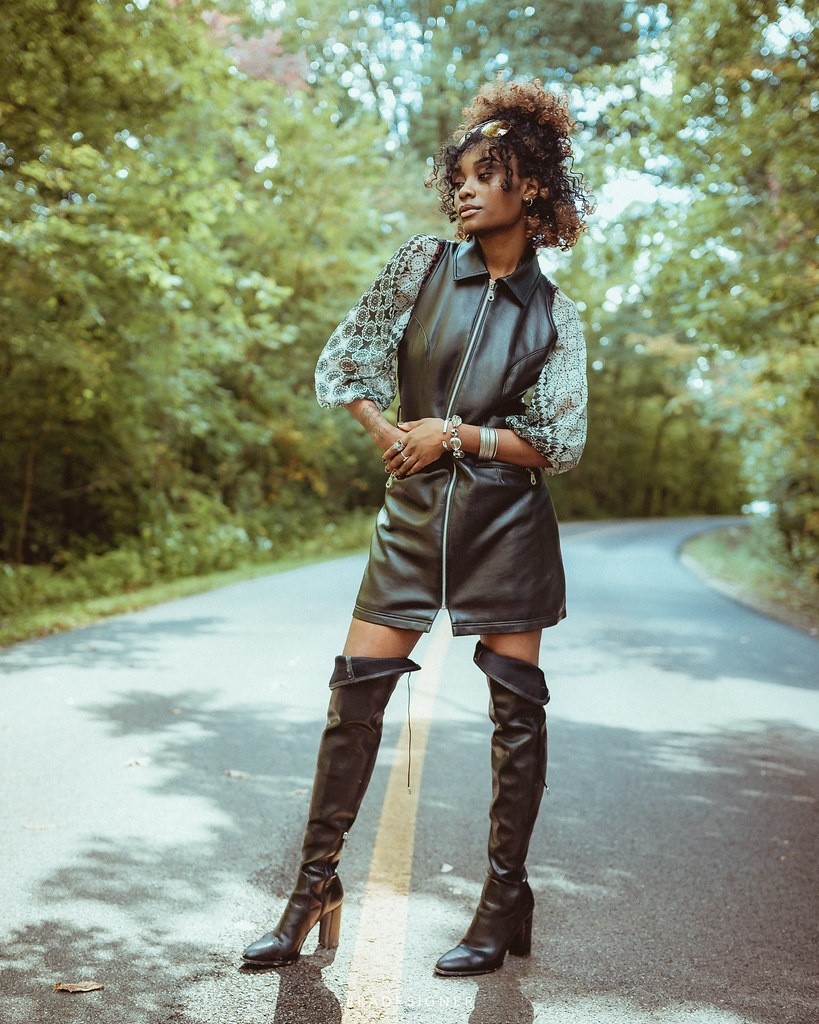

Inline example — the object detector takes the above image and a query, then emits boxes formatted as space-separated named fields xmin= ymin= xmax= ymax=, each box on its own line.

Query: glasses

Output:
xmin=457 ymin=120 xmax=528 ymax=150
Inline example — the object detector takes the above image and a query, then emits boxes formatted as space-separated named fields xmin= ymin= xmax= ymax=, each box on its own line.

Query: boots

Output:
xmin=436 ymin=641 xmax=551 ymax=976
xmin=240 ymin=657 xmax=422 ymax=966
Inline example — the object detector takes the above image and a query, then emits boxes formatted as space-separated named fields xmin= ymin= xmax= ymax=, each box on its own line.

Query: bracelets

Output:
xmin=441 ymin=418 xmax=451 ymax=452
xmin=476 ymin=426 xmax=499 ymax=461
xmin=450 ymin=415 xmax=465 ymax=458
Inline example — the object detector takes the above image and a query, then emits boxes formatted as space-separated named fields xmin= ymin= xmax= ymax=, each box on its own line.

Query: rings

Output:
xmin=393 ymin=439 xmax=405 ymax=452
xmin=401 ymin=452 xmax=410 ymax=461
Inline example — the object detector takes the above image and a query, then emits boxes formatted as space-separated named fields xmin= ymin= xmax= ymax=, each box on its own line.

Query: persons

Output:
xmin=240 ymin=80 xmax=588 ymax=977
